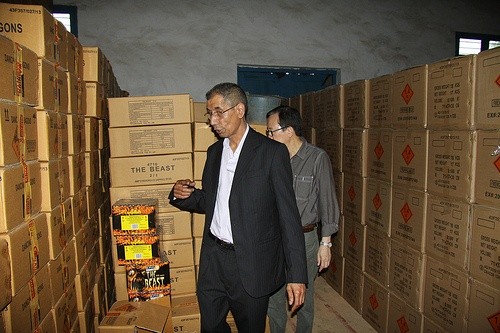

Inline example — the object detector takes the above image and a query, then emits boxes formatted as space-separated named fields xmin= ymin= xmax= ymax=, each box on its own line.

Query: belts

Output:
xmin=208 ymin=229 xmax=235 ymax=250
xmin=301 ymin=222 xmax=317 ymax=233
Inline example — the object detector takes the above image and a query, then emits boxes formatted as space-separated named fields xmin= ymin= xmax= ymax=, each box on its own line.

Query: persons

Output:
xmin=265 ymin=106 xmax=341 ymax=333
xmin=168 ymin=82 xmax=308 ymax=333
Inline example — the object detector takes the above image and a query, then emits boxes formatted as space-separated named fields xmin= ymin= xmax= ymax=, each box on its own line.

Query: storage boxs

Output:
xmin=0 ymin=3 xmax=500 ymax=333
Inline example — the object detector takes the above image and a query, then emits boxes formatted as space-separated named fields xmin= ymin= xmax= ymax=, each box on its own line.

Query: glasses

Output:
xmin=266 ymin=126 xmax=289 ymax=137
xmin=203 ymin=105 xmax=235 ymax=121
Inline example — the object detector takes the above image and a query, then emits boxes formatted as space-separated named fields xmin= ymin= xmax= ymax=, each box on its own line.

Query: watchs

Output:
xmin=319 ymin=241 xmax=333 ymax=248
